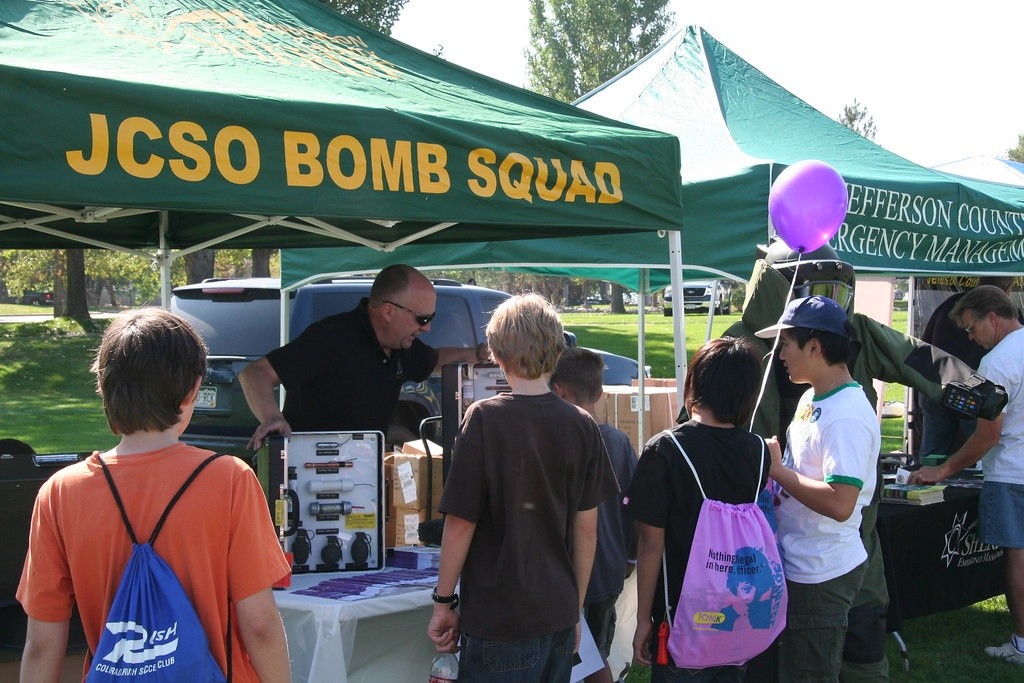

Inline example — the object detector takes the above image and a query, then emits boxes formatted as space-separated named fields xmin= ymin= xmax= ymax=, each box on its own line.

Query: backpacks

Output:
xmin=662 ymin=430 xmax=788 ymax=669
xmin=84 ymin=454 xmax=227 ymax=683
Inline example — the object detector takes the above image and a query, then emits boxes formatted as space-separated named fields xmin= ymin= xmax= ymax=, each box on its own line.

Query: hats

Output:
xmin=754 ymin=296 xmax=855 ymax=342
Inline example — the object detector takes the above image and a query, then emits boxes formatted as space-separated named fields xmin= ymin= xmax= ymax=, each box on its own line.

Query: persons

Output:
xmin=912 ymin=276 xmax=1018 ymax=456
xmin=546 ymin=345 xmax=638 ymax=683
xmin=623 ymin=336 xmax=771 ymax=682
xmin=908 ymin=286 xmax=1024 ymax=666
xmin=15 ymin=305 xmax=292 ymax=683
xmin=753 ymin=296 xmax=880 ymax=682
xmin=238 ymin=264 xmax=492 ymax=454
xmin=425 ymin=292 xmax=622 ymax=683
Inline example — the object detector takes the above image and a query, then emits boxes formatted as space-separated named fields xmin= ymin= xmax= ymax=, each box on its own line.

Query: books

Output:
xmin=290 ymin=545 xmax=441 ymax=603
xmin=880 ymin=483 xmax=947 ymax=506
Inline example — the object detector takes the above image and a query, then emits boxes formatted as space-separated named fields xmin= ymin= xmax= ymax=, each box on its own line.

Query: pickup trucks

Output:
xmin=10 ymin=288 xmax=53 ymax=305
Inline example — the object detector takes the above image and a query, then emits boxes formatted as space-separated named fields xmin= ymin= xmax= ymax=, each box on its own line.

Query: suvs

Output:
xmin=167 ymin=274 xmax=651 ymax=466
xmin=662 ymin=277 xmax=732 ymax=316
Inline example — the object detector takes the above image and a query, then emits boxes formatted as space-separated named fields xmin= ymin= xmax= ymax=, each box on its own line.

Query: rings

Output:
xmin=918 ymin=473 xmax=922 ymax=479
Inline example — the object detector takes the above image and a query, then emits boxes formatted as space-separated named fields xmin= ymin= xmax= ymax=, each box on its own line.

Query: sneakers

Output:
xmin=984 ymin=633 xmax=1024 ymax=665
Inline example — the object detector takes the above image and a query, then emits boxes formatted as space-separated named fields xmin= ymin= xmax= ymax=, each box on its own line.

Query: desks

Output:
xmin=869 ymin=470 xmax=1005 ymax=674
xmin=271 ymin=561 xmax=640 ymax=683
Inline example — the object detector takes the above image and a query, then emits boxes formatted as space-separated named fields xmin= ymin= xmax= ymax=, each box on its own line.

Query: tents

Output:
xmin=0 ymin=0 xmax=690 ymax=683
xmin=276 ymin=21 xmax=1024 ymax=429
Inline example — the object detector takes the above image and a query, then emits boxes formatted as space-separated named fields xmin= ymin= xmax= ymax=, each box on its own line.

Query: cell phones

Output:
xmin=941 ymin=382 xmax=984 ymax=418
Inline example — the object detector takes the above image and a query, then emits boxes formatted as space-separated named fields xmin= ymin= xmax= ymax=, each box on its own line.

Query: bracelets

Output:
xmin=431 ymin=587 xmax=459 ymax=611
xmin=475 ymin=342 xmax=491 ymax=365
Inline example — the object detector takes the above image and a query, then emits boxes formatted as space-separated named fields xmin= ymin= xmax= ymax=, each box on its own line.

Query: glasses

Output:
xmin=964 ymin=319 xmax=978 ymax=334
xmin=382 ymin=300 xmax=436 ymax=326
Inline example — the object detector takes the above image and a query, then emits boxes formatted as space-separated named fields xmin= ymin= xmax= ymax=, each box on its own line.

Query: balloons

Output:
xmin=769 ymin=159 xmax=849 ymax=254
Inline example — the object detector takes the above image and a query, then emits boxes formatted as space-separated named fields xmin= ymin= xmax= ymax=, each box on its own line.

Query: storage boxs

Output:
xmin=600 ymin=377 xmax=680 ymax=459
xmin=383 ymin=438 xmax=444 ymax=548
